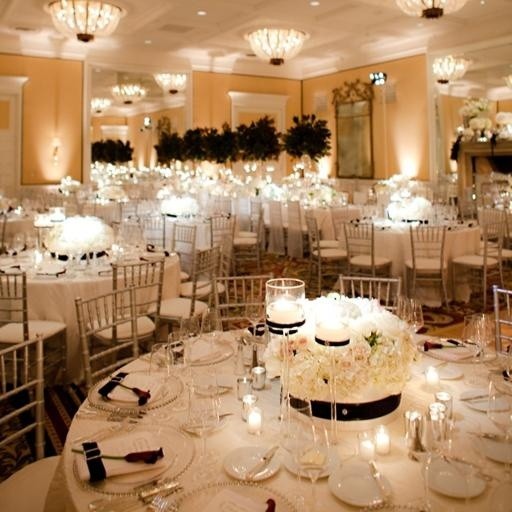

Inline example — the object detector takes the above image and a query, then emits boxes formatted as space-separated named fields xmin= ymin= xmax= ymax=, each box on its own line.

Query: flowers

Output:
xmin=456 ymin=96 xmax=512 ymax=145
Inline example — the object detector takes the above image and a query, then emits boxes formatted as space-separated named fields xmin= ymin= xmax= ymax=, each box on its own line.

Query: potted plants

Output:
xmin=181 ymin=113 xmax=333 ymax=176
xmin=91 ymin=131 xmax=184 ymax=167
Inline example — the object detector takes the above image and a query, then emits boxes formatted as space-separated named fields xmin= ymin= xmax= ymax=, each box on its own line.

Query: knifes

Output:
xmin=245 ymin=446 xmax=280 ymax=479
xmin=86 ymin=476 xmax=183 ymax=512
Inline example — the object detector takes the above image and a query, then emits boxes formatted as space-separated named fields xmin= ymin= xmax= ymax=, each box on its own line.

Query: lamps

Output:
xmin=42 ymin=0 xmax=128 ymax=45
xmin=90 ymin=97 xmax=112 ymax=115
xmin=107 ymin=85 xmax=150 ymax=105
xmin=431 ymin=55 xmax=473 ymax=84
xmin=153 ymin=71 xmax=189 ymax=96
xmin=244 ymin=28 xmax=310 ymax=68
xmin=392 ymin=0 xmax=467 ymax=22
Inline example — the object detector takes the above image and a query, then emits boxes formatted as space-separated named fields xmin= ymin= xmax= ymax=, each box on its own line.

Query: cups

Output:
xmin=246 ymin=408 xmax=262 ymax=436
xmin=249 ymin=366 xmax=267 ymax=391
xmin=374 ymin=425 xmax=391 ymax=456
xmin=243 ymin=393 xmax=259 ymax=422
xmin=358 ymin=431 xmax=376 ymax=462
xmin=236 ymin=375 xmax=252 ymax=400
xmin=405 ymin=411 xmax=424 ymax=448
xmin=427 ymin=404 xmax=449 ymax=450
xmin=433 ymin=390 xmax=452 ymax=428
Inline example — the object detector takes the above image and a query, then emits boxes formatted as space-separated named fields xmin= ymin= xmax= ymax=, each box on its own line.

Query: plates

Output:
xmin=165 ymin=481 xmax=296 ymax=512
xmin=422 ymin=457 xmax=488 ymax=499
xmin=463 ymin=313 xmax=492 ymax=383
xmin=424 ymin=363 xmax=465 ymax=381
xmin=461 ymin=387 xmax=510 ymax=412
xmin=280 ymin=429 xmax=343 ymax=477
xmin=468 ymin=417 xmax=511 ymax=464
xmin=72 ymin=423 xmax=194 ymax=494
xmin=87 ymin=370 xmax=185 ymax=415
xmin=224 ymin=445 xmax=281 ymax=483
xmin=186 ymin=333 xmax=236 ymax=365
xmin=416 ymin=336 xmax=498 ymax=364
xmin=328 ymin=462 xmax=391 ymax=505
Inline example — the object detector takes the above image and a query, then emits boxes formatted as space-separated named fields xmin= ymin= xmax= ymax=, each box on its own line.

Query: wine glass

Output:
xmin=294 ymin=422 xmax=329 ymax=508
xmin=178 ymin=313 xmax=200 ymax=378
xmin=10 ymin=194 xmax=49 ymax=217
xmin=244 ymin=295 xmax=265 ymax=352
xmin=188 ymin=388 xmax=219 ymax=482
xmin=138 ymin=199 xmax=160 ymax=216
xmin=200 ymin=309 xmax=224 ymax=376
xmin=277 ymin=190 xmax=349 ymax=208
xmin=148 ymin=343 xmax=175 ymax=420
xmin=426 ymin=202 xmax=458 ymax=225
xmin=391 ymin=295 xmax=411 ymax=323
xmin=477 ymin=188 xmax=512 ymax=209
xmin=1 ymin=227 xmax=51 ymax=273
xmin=489 ymin=377 xmax=512 ymax=481
xmin=409 ymin=419 xmax=445 ymax=510
xmin=167 ymin=330 xmax=186 ymax=377
xmin=111 ymin=221 xmax=147 ymax=266
xmin=264 ymin=277 xmax=308 ymax=453
xmin=314 ymin=302 xmax=357 ymax=458
xmin=402 ymin=300 xmax=423 ymax=362
xmin=278 ymin=398 xmax=315 ymax=509
xmin=446 ymin=417 xmax=487 ymax=511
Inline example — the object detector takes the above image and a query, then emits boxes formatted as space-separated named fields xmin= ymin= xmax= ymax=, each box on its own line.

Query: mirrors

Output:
xmin=89 ymin=63 xmax=190 ymax=170
